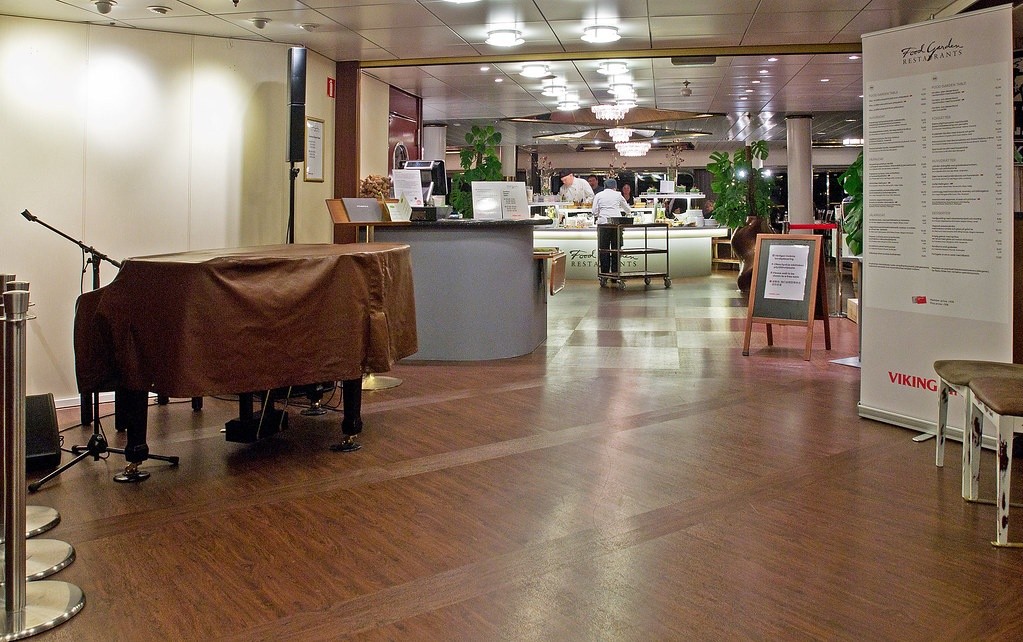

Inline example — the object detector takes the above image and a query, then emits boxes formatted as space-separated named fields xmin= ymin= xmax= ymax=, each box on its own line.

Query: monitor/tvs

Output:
xmin=400 ymin=159 xmax=448 ymax=195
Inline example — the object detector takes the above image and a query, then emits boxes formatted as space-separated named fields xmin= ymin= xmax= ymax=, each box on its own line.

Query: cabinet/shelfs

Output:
xmin=598 ymin=223 xmax=669 ymax=290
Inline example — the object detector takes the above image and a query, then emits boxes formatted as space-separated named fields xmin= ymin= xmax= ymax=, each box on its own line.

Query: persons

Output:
xmin=588 ymin=175 xmax=604 ymax=195
xmin=665 ymin=198 xmax=685 ymax=219
xmin=704 ymin=200 xmax=714 ymax=219
xmin=592 ymin=179 xmax=631 ymax=283
xmin=559 ymin=168 xmax=594 ymax=217
xmin=621 ymin=182 xmax=634 ymax=205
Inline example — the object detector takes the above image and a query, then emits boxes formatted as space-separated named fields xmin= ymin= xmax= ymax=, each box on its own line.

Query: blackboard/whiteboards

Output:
xmin=747 ymin=234 xmax=829 ymax=326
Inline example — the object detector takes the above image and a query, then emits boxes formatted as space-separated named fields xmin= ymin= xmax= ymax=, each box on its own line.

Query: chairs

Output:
xmin=934 ymin=360 xmax=1023 ymax=547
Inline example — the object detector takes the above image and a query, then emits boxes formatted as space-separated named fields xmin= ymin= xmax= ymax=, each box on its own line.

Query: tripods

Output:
xmin=21 ymin=210 xmax=179 ymax=492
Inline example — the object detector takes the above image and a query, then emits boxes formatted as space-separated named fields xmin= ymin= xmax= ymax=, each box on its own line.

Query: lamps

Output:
xmin=681 ymin=82 xmax=691 ymax=97
xmin=486 ymin=27 xmax=651 ymax=156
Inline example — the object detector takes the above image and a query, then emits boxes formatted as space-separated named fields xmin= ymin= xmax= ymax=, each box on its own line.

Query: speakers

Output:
xmin=25 ymin=393 xmax=61 ymax=470
xmin=287 ymin=47 xmax=307 ymax=162
